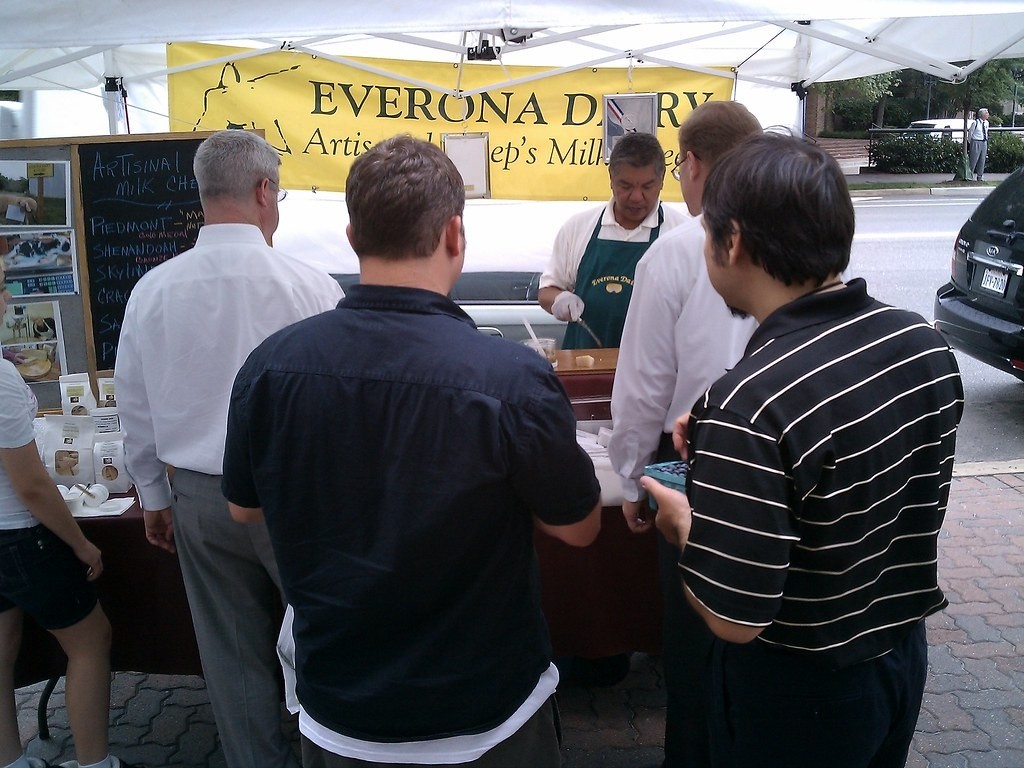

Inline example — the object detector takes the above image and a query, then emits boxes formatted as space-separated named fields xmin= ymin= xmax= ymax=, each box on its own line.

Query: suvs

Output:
xmin=934 ymin=163 xmax=1024 ymax=381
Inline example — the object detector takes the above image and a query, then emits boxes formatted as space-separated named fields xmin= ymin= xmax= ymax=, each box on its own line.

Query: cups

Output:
xmin=528 ymin=339 xmax=557 ymax=369
xmin=68 ymin=484 xmax=89 ymax=503
xmin=64 ymin=492 xmax=86 ymax=513
xmin=85 ymin=484 xmax=109 ymax=507
xmin=56 ymin=485 xmax=69 ymax=498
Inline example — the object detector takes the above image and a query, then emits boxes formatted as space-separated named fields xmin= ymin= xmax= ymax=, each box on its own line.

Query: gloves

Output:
xmin=550 ymin=291 xmax=585 ymax=323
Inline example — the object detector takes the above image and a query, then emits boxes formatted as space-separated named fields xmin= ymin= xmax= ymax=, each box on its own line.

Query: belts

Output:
xmin=974 ymin=139 xmax=988 ymax=141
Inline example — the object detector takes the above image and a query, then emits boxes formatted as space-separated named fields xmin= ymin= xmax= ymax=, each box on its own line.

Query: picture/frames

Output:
xmin=603 ymin=93 xmax=658 ymax=164
xmin=440 ymin=132 xmax=492 ymax=200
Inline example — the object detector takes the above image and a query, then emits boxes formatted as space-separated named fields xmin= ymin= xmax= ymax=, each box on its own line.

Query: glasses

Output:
xmin=260 ymin=177 xmax=288 ymax=202
xmin=671 ymin=152 xmax=702 ymax=181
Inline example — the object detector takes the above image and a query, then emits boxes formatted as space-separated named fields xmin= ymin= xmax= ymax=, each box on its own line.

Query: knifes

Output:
xmin=576 ymin=317 xmax=601 ymax=347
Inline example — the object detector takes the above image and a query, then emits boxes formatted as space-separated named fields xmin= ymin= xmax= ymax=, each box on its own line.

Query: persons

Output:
xmin=0 ymin=258 xmax=133 ymax=768
xmin=537 ymin=132 xmax=694 ymax=350
xmin=640 ymin=131 xmax=964 ymax=768
xmin=609 ymin=101 xmax=764 ymax=768
xmin=112 ymin=129 xmax=346 ymax=768
xmin=221 ymin=135 xmax=602 ymax=768
xmin=30 ymin=312 xmax=57 ymax=339
xmin=968 ymin=108 xmax=990 ymax=181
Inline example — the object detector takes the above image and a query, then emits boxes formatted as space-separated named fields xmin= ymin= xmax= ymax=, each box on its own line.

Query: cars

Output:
xmin=333 ymin=273 xmax=570 ymax=352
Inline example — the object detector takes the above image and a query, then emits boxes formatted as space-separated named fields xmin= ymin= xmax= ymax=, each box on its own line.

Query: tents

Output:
xmin=0 ymin=0 xmax=1024 ymax=142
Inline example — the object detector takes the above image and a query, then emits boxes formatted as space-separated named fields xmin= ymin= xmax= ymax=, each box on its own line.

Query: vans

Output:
xmin=901 ymin=118 xmax=976 ymax=143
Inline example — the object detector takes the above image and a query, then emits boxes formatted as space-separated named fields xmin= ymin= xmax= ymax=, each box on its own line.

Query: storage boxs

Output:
xmin=646 ymin=460 xmax=686 ymax=510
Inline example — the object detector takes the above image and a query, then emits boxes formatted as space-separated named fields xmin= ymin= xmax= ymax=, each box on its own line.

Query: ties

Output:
xmin=982 ymin=122 xmax=986 ymax=141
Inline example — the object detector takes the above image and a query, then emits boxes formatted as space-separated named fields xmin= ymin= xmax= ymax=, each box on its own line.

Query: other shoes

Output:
xmin=110 ymin=755 xmax=147 ymax=768
xmin=27 ymin=755 xmax=78 ymax=768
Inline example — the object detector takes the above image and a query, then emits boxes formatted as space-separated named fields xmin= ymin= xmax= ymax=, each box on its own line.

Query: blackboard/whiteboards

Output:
xmin=73 ymin=128 xmax=266 ymax=378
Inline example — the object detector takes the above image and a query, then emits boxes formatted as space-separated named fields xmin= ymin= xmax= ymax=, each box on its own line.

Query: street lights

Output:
xmin=1011 ymin=67 xmax=1023 ymax=127
xmin=922 ymin=71 xmax=939 ymax=119
